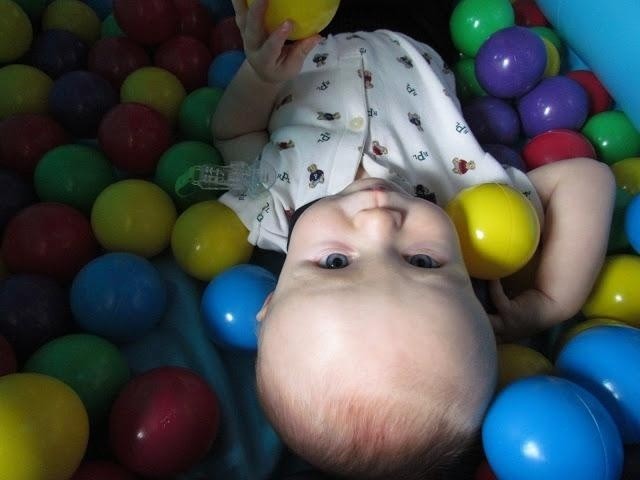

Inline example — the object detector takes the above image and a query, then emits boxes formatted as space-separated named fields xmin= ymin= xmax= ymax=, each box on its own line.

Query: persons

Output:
xmin=211 ymin=0 xmax=616 ymax=479
